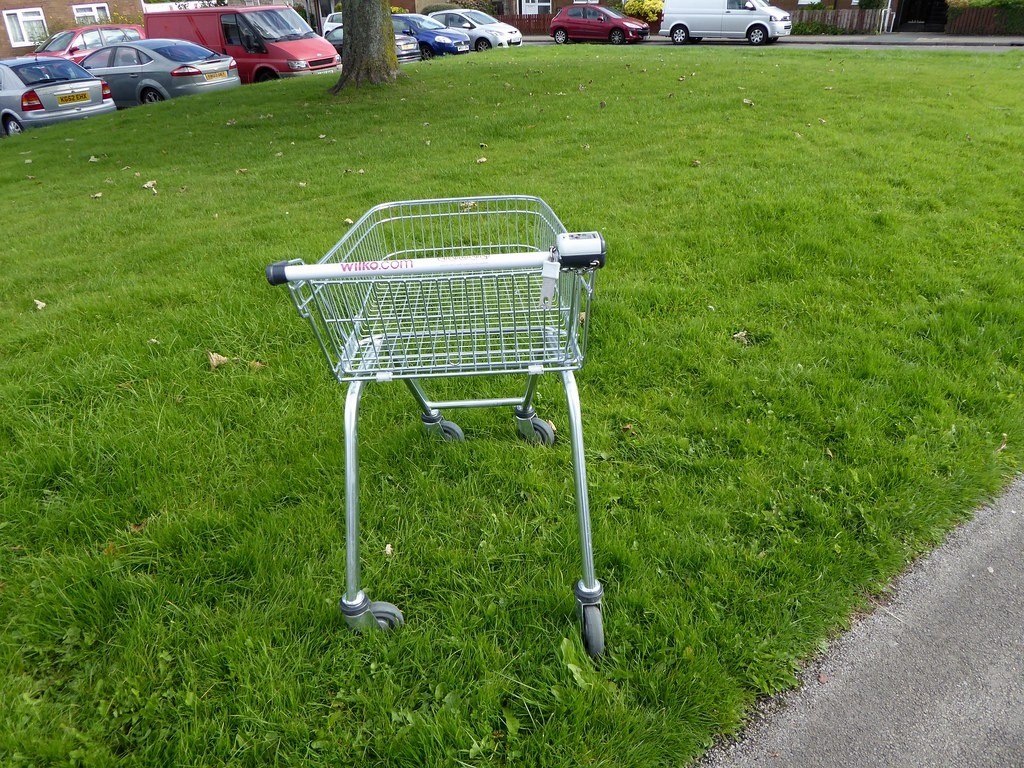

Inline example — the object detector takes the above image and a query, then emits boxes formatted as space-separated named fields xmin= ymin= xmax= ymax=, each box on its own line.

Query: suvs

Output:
xmin=324 ymin=13 xmax=343 ymax=38
xmin=20 ymin=23 xmax=146 ymax=81
xmin=549 ymin=4 xmax=650 ymax=45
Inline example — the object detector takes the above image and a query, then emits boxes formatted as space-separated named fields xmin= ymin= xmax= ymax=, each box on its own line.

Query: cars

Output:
xmin=429 ymin=8 xmax=523 ymax=51
xmin=323 ymin=24 xmax=422 ymax=67
xmin=387 ymin=13 xmax=472 ymax=61
xmin=0 ymin=56 xmax=118 ymax=136
xmin=79 ymin=38 xmax=242 ymax=109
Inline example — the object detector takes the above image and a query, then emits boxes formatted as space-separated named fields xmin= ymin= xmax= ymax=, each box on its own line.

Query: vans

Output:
xmin=144 ymin=3 xmax=342 ymax=84
xmin=658 ymin=0 xmax=793 ymax=45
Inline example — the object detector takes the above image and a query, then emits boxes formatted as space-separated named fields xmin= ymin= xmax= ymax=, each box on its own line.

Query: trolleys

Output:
xmin=263 ymin=193 xmax=608 ymax=658
